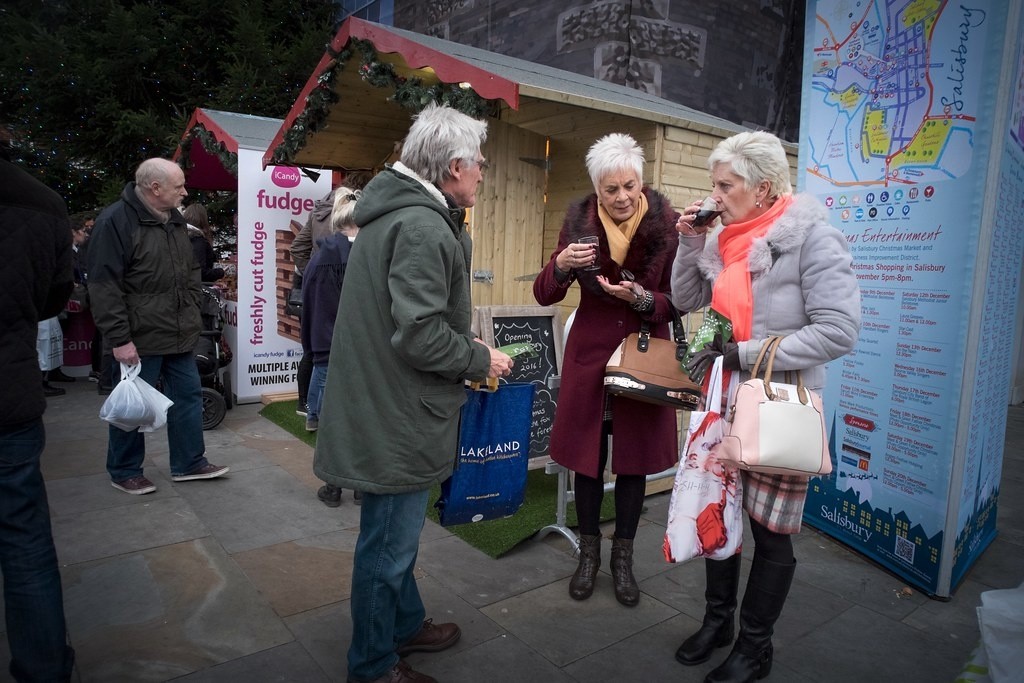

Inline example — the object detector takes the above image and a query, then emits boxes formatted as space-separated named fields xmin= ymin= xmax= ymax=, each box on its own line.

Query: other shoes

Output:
xmin=352 ymin=490 xmax=365 ymax=506
xmin=305 ymin=418 xmax=319 ymax=433
xmin=88 ymin=371 xmax=100 ymax=384
xmin=47 ymin=368 xmax=75 ymax=382
xmin=317 ymin=484 xmax=344 ymax=508
xmin=41 ymin=380 xmax=65 ymax=396
xmin=294 ymin=405 xmax=308 ymax=417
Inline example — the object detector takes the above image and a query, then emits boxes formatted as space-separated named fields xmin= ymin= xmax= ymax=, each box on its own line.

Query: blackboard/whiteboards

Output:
xmin=471 ymin=304 xmax=566 ymax=471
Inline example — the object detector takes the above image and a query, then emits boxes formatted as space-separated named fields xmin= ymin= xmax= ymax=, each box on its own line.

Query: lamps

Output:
xmin=298 ymin=165 xmax=321 ymax=184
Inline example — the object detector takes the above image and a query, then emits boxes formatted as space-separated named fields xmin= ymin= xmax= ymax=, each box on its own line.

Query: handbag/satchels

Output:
xmin=36 ymin=316 xmax=65 ymax=370
xmin=98 ymin=358 xmax=174 ymax=434
xmin=658 ymin=356 xmax=750 ymax=566
xmin=435 ymin=371 xmax=536 ymax=528
xmin=213 ymin=262 xmax=237 ymax=277
xmin=601 ymin=295 xmax=707 ymax=415
xmin=284 ymin=288 xmax=306 ymax=317
xmin=719 ymin=336 xmax=833 ymax=477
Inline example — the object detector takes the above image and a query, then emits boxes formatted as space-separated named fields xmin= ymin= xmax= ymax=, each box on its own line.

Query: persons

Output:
xmin=41 ymin=211 xmax=116 ymax=395
xmin=313 ymin=107 xmax=514 ymax=683
xmin=301 ymin=185 xmax=360 ymax=508
xmin=0 ymin=157 xmax=75 ymax=683
xmin=291 ymin=168 xmax=373 ymax=431
xmin=670 ymin=131 xmax=862 ymax=683
xmin=533 ymin=133 xmax=688 ymax=606
xmin=183 ymin=202 xmax=227 ymax=387
xmin=86 ymin=158 xmax=230 ymax=495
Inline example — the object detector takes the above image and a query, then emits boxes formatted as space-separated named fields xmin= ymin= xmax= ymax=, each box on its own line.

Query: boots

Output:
xmin=674 ymin=556 xmax=744 ymax=665
xmin=696 ymin=553 xmax=796 ymax=683
xmin=566 ymin=534 xmax=607 ymax=601
xmin=606 ymin=532 xmax=643 ymax=606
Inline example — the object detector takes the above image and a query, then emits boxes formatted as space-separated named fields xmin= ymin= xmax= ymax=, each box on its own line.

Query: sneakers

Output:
xmin=171 ymin=460 xmax=231 ymax=482
xmin=110 ymin=475 xmax=158 ymax=495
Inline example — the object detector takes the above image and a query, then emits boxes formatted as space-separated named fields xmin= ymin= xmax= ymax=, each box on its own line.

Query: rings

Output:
xmin=610 ymin=292 xmax=615 ymax=295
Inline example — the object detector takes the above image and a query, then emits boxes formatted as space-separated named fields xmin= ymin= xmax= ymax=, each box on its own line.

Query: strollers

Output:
xmin=201 ymin=282 xmax=228 ymax=431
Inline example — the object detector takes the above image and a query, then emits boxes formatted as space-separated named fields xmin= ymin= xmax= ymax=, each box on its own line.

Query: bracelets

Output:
xmin=633 ymin=291 xmax=652 ymax=311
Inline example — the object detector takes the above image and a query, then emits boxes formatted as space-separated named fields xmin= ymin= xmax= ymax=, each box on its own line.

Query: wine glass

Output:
xmin=578 ymin=236 xmax=601 ymax=271
xmin=683 ymin=196 xmax=725 ymax=236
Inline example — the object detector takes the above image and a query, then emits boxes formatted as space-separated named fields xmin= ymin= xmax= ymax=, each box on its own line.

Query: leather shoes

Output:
xmin=361 ymin=660 xmax=437 ymax=682
xmin=392 ymin=616 xmax=463 ymax=659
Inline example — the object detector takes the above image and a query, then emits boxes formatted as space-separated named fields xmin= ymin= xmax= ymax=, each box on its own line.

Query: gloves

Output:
xmin=684 ymin=341 xmax=716 ymax=387
xmin=703 ymin=340 xmax=741 ymax=372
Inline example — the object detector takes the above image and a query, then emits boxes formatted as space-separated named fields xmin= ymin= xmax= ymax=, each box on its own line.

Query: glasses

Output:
xmin=619 ymin=267 xmax=646 ymax=302
xmin=470 ymin=158 xmax=493 ymax=173
